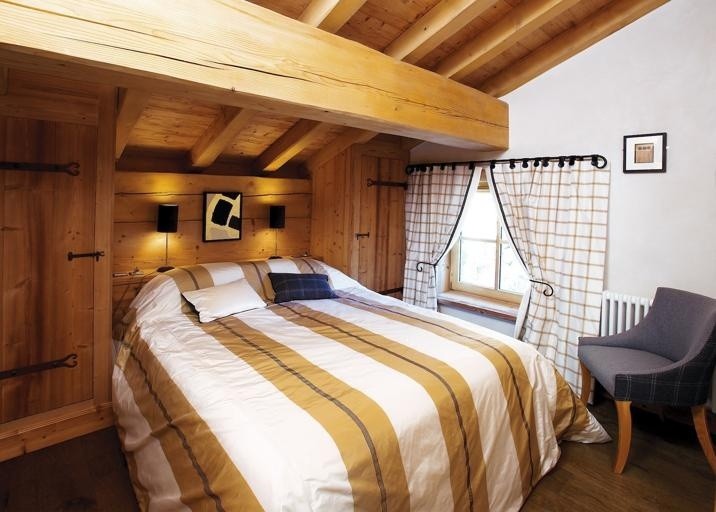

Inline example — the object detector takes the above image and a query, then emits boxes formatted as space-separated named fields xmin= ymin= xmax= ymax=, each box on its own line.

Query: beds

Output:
xmin=110 ymin=259 xmax=611 ymax=512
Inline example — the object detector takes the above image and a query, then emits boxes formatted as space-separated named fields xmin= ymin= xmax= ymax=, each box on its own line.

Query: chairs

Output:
xmin=578 ymin=286 xmax=716 ymax=477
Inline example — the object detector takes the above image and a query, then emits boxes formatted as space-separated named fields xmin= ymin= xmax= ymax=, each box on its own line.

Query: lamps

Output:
xmin=157 ymin=203 xmax=179 ymax=273
xmin=269 ymin=206 xmax=285 ymax=259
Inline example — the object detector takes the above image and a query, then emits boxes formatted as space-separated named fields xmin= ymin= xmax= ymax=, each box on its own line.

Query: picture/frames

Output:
xmin=623 ymin=132 xmax=667 ymax=173
xmin=202 ymin=190 xmax=242 ymax=242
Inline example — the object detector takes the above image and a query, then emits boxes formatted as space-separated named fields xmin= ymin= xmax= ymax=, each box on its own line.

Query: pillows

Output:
xmin=268 ymin=272 xmax=337 ymax=303
xmin=181 ymin=278 xmax=267 ymax=323
